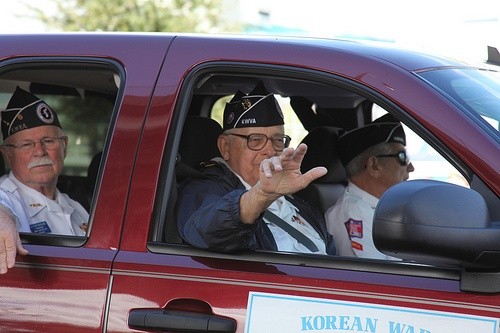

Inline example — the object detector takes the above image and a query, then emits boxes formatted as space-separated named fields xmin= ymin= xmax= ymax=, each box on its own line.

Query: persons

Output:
xmin=324 ymin=112 xmax=414 ymax=261
xmin=174 ymin=79 xmax=337 ymax=256
xmin=0 ymin=84 xmax=90 ymax=275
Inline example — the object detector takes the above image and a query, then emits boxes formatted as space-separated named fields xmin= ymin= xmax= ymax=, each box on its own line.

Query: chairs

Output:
xmin=164 ymin=115 xmax=226 ymax=242
xmin=295 ymin=125 xmax=354 ymax=219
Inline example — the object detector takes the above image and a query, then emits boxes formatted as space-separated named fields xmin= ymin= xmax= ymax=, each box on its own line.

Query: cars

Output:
xmin=0 ymin=31 xmax=499 ymax=333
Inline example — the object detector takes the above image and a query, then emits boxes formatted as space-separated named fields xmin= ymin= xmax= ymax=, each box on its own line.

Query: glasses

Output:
xmin=376 ymin=150 xmax=407 ymax=165
xmin=220 ymin=133 xmax=291 ymax=152
xmin=2 ymin=138 xmax=64 ymax=153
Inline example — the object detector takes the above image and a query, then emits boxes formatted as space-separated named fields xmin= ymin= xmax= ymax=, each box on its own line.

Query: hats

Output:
xmin=0 ymin=85 xmax=62 ymax=140
xmin=223 ymin=81 xmax=284 ymax=129
xmin=337 ymin=111 xmax=406 ymax=167
